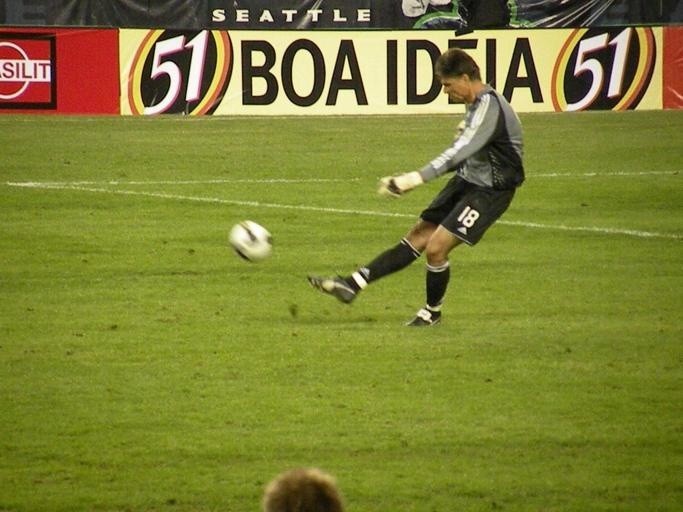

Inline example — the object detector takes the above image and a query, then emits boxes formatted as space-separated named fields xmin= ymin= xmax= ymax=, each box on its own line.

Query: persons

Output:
xmin=262 ymin=468 xmax=345 ymax=512
xmin=305 ymin=49 xmax=526 ymax=326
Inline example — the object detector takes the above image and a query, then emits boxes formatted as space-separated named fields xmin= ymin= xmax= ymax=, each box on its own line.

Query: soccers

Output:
xmin=228 ymin=219 xmax=274 ymax=263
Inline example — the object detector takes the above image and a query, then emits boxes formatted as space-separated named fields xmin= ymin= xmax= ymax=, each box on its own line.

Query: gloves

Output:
xmin=378 ymin=172 xmax=422 ymax=197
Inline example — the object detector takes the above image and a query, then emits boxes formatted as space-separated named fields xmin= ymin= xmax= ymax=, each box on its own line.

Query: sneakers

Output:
xmin=307 ymin=272 xmax=356 ymax=304
xmin=406 ymin=307 xmax=442 ymax=326
